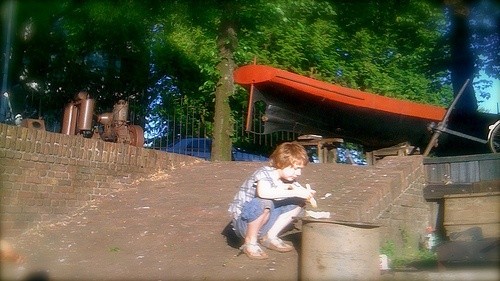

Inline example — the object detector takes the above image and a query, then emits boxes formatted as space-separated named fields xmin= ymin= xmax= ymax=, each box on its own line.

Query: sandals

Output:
xmin=241 ymin=243 xmax=269 ymax=259
xmin=260 ymin=236 xmax=292 ymax=252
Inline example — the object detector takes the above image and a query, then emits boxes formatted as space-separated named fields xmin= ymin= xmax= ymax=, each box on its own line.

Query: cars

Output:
xmin=157 ymin=137 xmax=270 ymax=162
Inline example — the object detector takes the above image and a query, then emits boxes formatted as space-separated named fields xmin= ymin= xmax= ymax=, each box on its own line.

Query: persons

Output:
xmin=229 ymin=143 xmax=316 ymax=259
